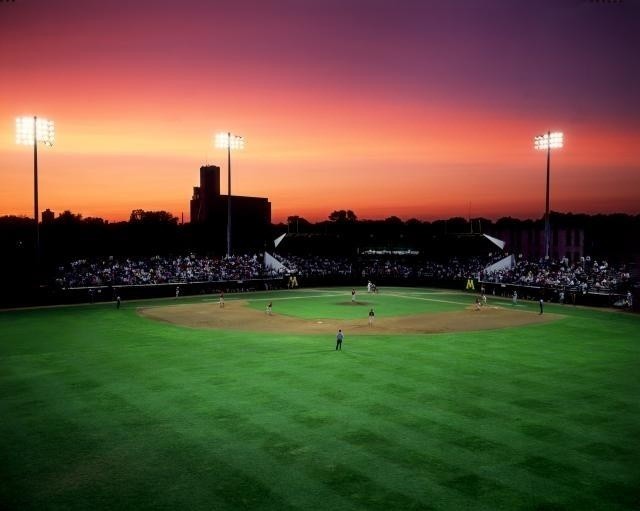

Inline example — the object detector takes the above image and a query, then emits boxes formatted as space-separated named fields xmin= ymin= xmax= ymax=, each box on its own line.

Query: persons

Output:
xmin=117 ymin=295 xmax=120 ymax=310
xmin=220 ymin=290 xmax=225 ymax=308
xmin=176 ymin=284 xmax=180 ymax=298
xmin=55 ymin=248 xmax=633 ymax=294
xmin=558 ymin=290 xmax=565 ymax=305
xmin=476 ymin=293 xmax=487 ymax=309
xmin=540 ymin=298 xmax=544 ymax=313
xmin=624 ymin=291 xmax=633 ymax=309
xmin=268 ymin=301 xmax=272 ymax=312
xmin=352 ymin=288 xmax=356 ymax=303
xmin=512 ymin=289 xmax=517 ymax=306
xmin=335 ymin=330 xmax=344 ymax=351
xmin=368 ymin=309 xmax=374 ymax=329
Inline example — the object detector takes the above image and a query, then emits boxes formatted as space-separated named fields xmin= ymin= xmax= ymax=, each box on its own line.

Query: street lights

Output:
xmin=214 ymin=130 xmax=245 ymax=254
xmin=15 ymin=115 xmax=55 ymax=245
xmin=534 ymin=130 xmax=564 ymax=259
